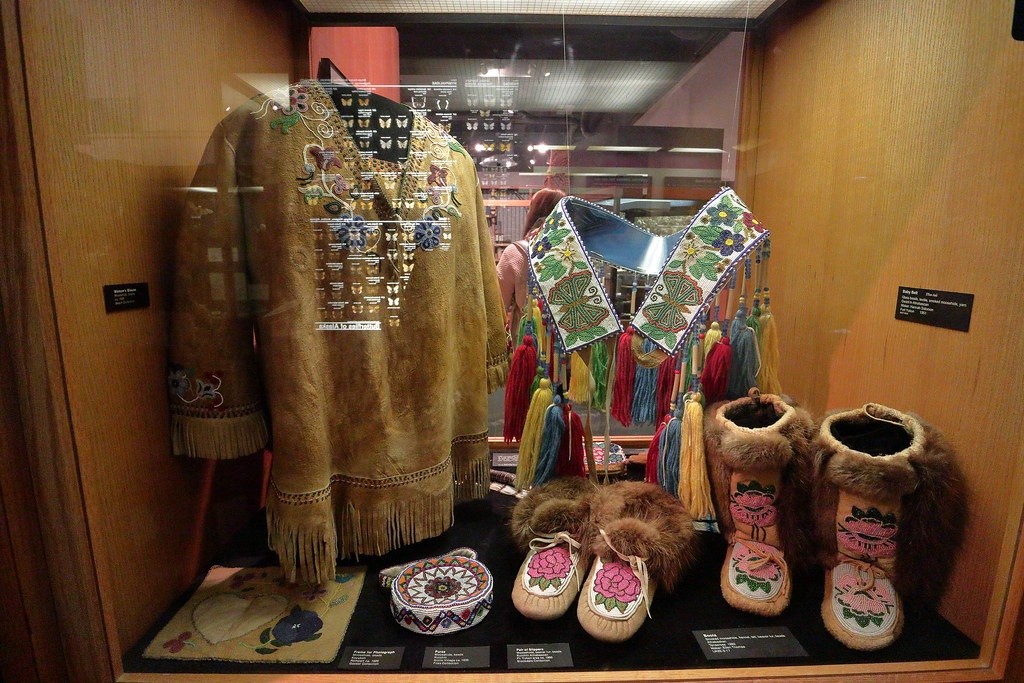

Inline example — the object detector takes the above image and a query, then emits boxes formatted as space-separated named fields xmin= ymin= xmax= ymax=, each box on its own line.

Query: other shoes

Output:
xmin=511 ymin=476 xmax=695 ymax=641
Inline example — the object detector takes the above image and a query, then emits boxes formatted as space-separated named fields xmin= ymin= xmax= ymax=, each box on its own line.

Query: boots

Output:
xmin=702 ymin=386 xmax=811 ymax=617
xmin=811 ymin=404 xmax=963 ymax=649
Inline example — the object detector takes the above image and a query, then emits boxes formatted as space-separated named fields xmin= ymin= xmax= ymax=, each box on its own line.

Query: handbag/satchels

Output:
xmin=582 ymin=442 xmax=628 ymax=476
xmin=379 ymin=548 xmax=493 ymax=635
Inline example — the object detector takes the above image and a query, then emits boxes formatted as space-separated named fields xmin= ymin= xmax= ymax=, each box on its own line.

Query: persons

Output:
xmin=496 ymin=188 xmax=566 ymax=355
